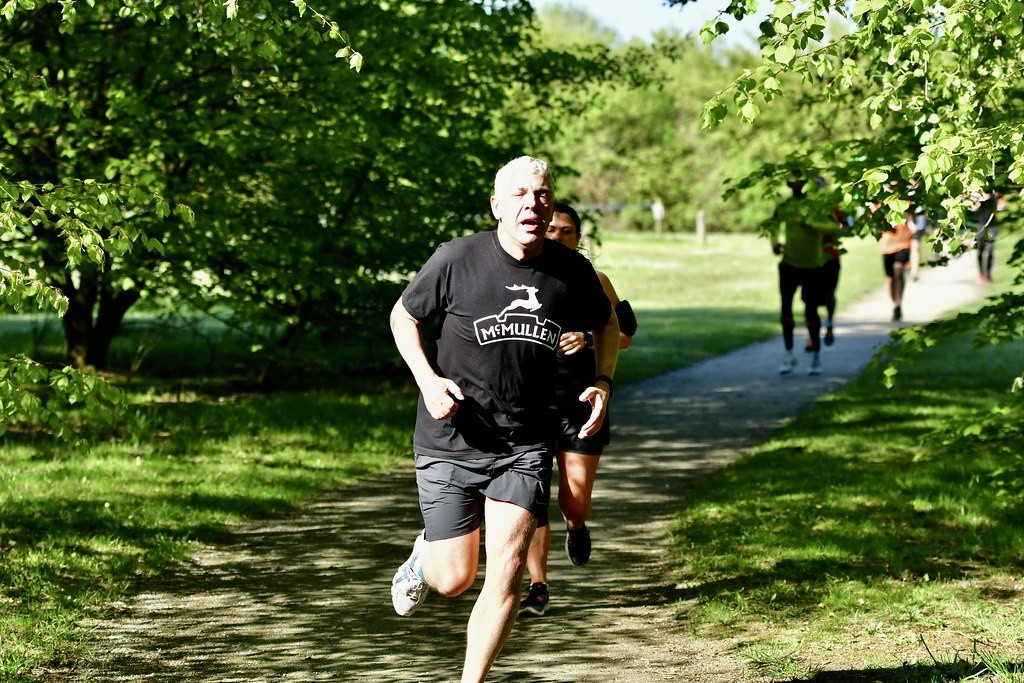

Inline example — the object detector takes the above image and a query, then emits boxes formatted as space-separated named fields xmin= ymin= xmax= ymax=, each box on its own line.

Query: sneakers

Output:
xmin=391 ymin=528 xmax=431 ymax=616
xmin=824 ymin=332 xmax=834 ymax=345
xmin=779 ymin=357 xmax=799 ymax=377
xmin=561 ymin=511 xmax=592 ymax=567
xmin=809 ymin=362 xmax=823 ymax=374
xmin=518 ymin=581 xmax=552 ymax=615
xmin=805 ymin=338 xmax=812 ymax=351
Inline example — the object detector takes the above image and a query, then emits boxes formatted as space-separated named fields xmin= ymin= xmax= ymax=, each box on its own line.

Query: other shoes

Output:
xmin=893 ymin=309 xmax=901 ymax=321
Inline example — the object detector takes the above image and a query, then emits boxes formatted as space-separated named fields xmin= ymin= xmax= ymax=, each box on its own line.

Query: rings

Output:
xmin=570 ymin=344 xmax=574 ymax=348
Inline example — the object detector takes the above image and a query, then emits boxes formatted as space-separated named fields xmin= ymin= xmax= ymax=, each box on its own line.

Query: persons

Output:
xmin=390 ymin=156 xmax=620 ymax=683
xmin=871 ymin=179 xmax=918 ymax=321
xmin=770 ymin=170 xmax=836 ymax=375
xmin=905 ymin=177 xmax=926 ymax=280
xmin=517 ymin=204 xmax=639 ymax=616
xmin=976 ymin=196 xmax=997 ymax=284
xmin=801 ymin=177 xmax=847 ymax=353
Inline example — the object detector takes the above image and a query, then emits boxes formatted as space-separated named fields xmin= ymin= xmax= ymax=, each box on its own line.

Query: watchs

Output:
xmin=582 ymin=331 xmax=594 ymax=348
xmin=596 ymin=375 xmax=614 ymax=398
